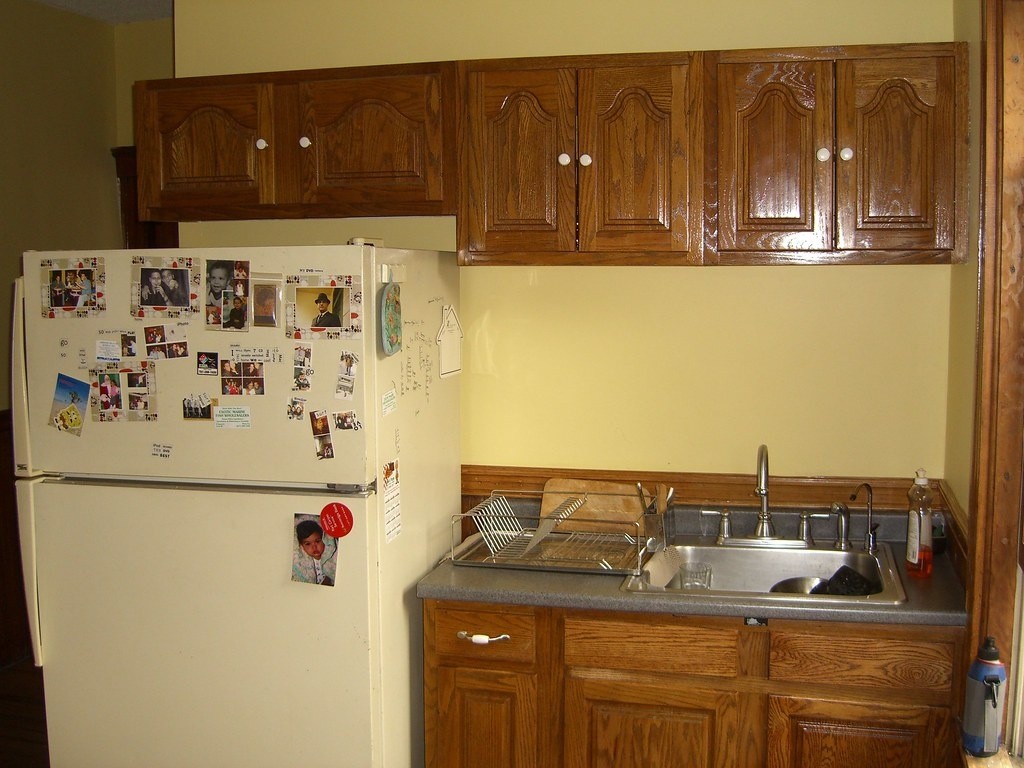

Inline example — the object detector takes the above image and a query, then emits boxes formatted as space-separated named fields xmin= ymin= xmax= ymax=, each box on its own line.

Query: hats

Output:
xmin=315 ymin=293 xmax=330 ymax=304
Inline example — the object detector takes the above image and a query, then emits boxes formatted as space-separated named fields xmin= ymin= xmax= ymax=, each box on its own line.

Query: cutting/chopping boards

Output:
xmin=538 ymin=478 xmax=651 ymax=537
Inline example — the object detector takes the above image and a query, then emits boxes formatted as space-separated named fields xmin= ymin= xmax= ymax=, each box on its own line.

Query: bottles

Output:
xmin=905 ymin=469 xmax=933 ymax=578
xmin=962 ymin=635 xmax=1007 ymax=758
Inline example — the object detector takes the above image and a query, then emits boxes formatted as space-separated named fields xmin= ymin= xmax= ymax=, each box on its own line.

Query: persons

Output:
xmin=291 ymin=515 xmax=339 ymax=586
xmin=184 ymin=346 xmax=355 ymax=459
xmin=312 ymin=293 xmax=341 ymax=327
xmin=100 ymin=373 xmax=122 ymax=409
xmin=75 ymin=273 xmax=91 ymax=306
xmin=123 ymin=329 xmax=187 ymax=359
xmin=51 ymin=275 xmax=65 ymax=307
xmin=141 ymin=269 xmax=188 ymax=306
xmin=206 ymin=261 xmax=246 ymax=329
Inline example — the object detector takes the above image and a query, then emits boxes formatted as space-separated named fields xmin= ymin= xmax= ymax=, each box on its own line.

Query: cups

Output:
xmin=677 ymin=562 xmax=710 ymax=589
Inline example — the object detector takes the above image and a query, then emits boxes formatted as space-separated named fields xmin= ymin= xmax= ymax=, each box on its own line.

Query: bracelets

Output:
xmin=165 ymin=298 xmax=169 ymax=302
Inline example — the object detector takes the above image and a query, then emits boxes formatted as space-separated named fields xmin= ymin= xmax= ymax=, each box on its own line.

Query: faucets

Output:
xmin=849 ymin=482 xmax=880 ymax=551
xmin=756 ymin=446 xmax=774 ymax=537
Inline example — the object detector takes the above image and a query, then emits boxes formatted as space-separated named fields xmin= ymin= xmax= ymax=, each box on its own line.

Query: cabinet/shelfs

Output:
xmin=455 ymin=49 xmax=706 ymax=267
xmin=132 ymin=60 xmax=460 ymax=220
xmin=702 ymin=41 xmax=972 ymax=266
xmin=433 ymin=608 xmax=967 ymax=768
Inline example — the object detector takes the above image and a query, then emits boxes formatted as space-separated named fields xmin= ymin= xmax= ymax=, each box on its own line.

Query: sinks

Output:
xmin=622 ymin=535 xmax=906 ymax=602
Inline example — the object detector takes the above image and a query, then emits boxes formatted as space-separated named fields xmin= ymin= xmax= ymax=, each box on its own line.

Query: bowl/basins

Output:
xmin=769 ymin=575 xmax=829 ymax=594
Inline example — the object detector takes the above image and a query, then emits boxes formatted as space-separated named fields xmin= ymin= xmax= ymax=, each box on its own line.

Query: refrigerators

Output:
xmin=8 ymin=247 xmax=461 ymax=768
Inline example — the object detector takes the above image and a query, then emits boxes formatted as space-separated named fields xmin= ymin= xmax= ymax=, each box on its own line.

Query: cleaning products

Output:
xmin=907 ymin=469 xmax=933 ymax=578
